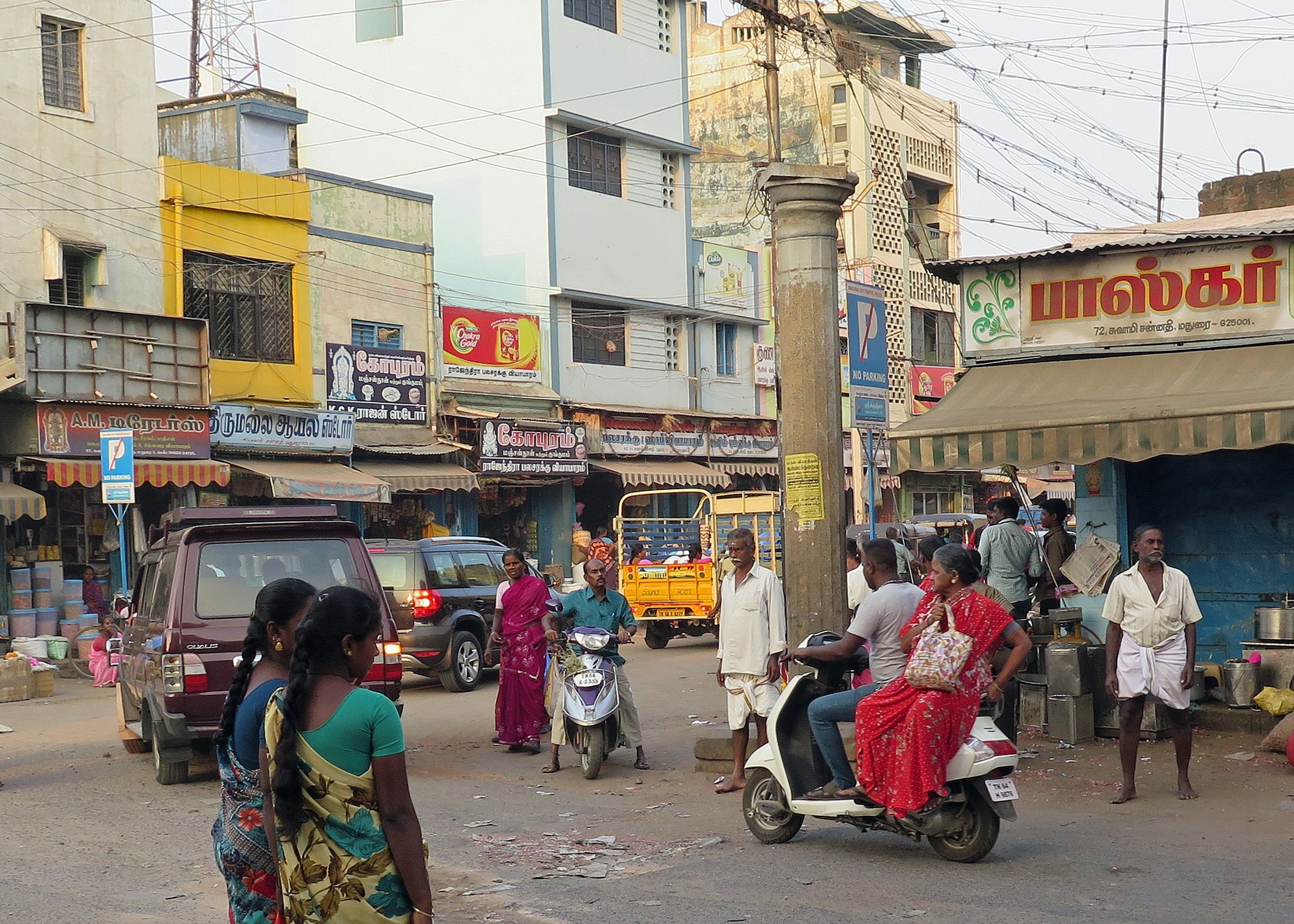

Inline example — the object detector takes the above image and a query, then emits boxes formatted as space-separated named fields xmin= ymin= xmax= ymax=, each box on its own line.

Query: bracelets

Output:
xmin=543 ymin=627 xmax=553 ymax=633
xmin=993 ymin=681 xmax=1005 ymax=693
xmin=413 ymin=904 xmax=436 ymax=919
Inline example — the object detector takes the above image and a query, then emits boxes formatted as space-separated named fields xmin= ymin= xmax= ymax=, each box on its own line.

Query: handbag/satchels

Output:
xmin=543 ymin=653 xmax=563 ymax=719
xmin=903 ymin=602 xmax=973 ymax=691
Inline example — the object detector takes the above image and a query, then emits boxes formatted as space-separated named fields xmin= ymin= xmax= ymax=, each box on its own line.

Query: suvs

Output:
xmin=366 ymin=535 xmax=569 ymax=693
xmin=105 ymin=506 xmax=400 ymax=783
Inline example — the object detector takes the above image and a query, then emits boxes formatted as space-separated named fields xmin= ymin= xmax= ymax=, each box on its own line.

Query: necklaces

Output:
xmin=305 ymin=671 xmax=350 ymax=682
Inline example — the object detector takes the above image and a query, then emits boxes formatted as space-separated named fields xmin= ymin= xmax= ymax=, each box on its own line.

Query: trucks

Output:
xmin=613 ymin=489 xmax=782 ymax=649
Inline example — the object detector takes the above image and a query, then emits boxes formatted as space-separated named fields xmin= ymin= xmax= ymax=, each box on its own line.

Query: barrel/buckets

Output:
xmin=1190 ymin=666 xmax=1208 ymax=702
xmin=1017 ymin=619 xmax=1027 ymax=634
xmin=1030 ymin=615 xmax=1053 ymax=635
xmin=8 ymin=567 xmax=101 ymax=659
xmin=1223 ymin=659 xmax=1263 ymax=707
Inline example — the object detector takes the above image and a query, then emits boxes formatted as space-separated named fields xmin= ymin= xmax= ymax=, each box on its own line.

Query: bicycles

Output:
xmin=67 ymin=624 xmax=103 ymax=678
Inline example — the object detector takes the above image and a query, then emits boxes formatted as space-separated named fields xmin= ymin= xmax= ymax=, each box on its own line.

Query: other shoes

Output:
xmin=542 ymin=759 xmax=560 ymax=772
xmin=539 ymin=722 xmax=550 ymax=734
xmin=524 ymin=741 xmax=541 ymax=754
xmin=803 ymin=781 xmax=840 ymax=798
xmin=492 ymin=737 xmax=499 ymax=744
xmin=509 ymin=743 xmax=528 ymax=752
xmin=634 ymin=759 xmax=650 ymax=769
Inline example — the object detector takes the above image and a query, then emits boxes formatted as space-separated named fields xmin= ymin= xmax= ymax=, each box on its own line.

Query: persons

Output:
xmin=843 ymin=518 xmax=966 ymax=616
xmin=588 ymin=526 xmax=622 ymax=594
xmin=1100 ymin=522 xmax=1204 ymax=806
xmin=1017 ymin=517 xmax=1033 ymax=531
xmin=708 ymin=555 xmax=735 ymax=620
xmin=965 ymin=497 xmax=1007 ymax=586
xmin=88 ymin=613 xmax=124 ymax=689
xmin=483 ymin=555 xmax=554 ymax=747
xmin=779 ymin=536 xmax=929 ymax=800
xmin=977 ymin=496 xmax=1043 ymax=629
xmin=259 ymin=558 xmax=284 ymax=586
xmin=833 ymin=541 xmax=1034 ymax=812
xmin=681 ymin=543 xmax=710 ymax=563
xmin=537 ymin=557 xmax=654 ymax=774
xmin=713 ymin=526 xmax=789 ymax=796
xmin=80 ymin=564 xmax=106 ymax=619
xmin=626 ymin=542 xmax=654 ymax=567
xmin=262 ymin=583 xmax=438 ymax=924
xmin=1033 ymin=495 xmax=1078 ymax=623
xmin=212 ymin=575 xmax=325 ymax=924
xmin=490 ymin=547 xmax=556 ymax=759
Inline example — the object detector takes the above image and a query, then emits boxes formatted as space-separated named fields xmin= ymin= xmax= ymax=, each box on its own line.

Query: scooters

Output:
xmin=742 ymin=629 xmax=1020 ymax=863
xmin=544 ymin=598 xmax=633 ymax=779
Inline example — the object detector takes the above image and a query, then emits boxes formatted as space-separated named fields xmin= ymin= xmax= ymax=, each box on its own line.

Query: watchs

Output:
xmin=625 ymin=630 xmax=632 ymax=637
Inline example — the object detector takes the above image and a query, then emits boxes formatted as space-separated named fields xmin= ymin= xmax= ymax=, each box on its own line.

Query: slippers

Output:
xmin=834 ymin=784 xmax=881 ymax=808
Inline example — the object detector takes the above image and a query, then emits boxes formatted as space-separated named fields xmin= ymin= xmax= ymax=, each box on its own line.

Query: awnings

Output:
xmin=586 ymin=452 xmax=736 ymax=493
xmin=216 ymin=455 xmax=395 ymax=505
xmin=702 ymin=459 xmax=779 ymax=479
xmin=341 ymin=456 xmax=484 ymax=497
xmin=843 ymin=472 xmax=901 ymax=493
xmin=15 ymin=453 xmax=231 ymax=488
xmin=0 ymin=479 xmax=47 ymax=522
xmin=883 ymin=341 xmax=1294 ymax=482
